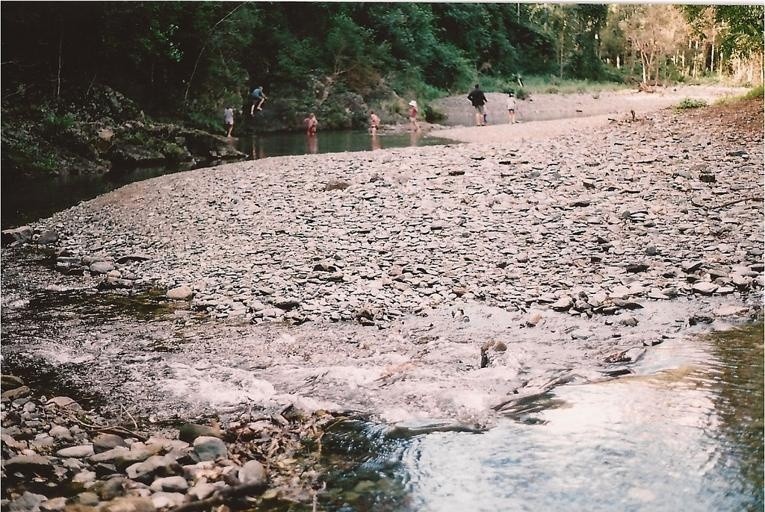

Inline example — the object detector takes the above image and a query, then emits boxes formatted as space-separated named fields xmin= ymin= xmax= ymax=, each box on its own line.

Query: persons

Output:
xmin=406 ymin=100 xmax=419 ymax=132
xmin=467 ymin=84 xmax=489 ymax=126
xmin=368 ymin=110 xmax=380 ymax=136
xmin=482 ymin=101 xmax=488 ymax=122
xmin=506 ymin=92 xmax=517 ymax=124
xmin=223 ymin=106 xmax=234 ymax=137
xmin=250 ymin=86 xmax=268 ymax=116
xmin=304 ymin=113 xmax=317 ymax=136
